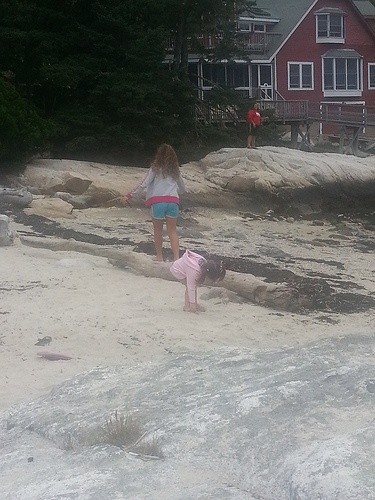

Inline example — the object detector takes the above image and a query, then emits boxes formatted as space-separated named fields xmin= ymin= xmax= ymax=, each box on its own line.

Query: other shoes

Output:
xmin=247 ymin=145 xmax=251 ymax=148
xmin=184 ymin=305 xmax=206 ymax=313
xmin=253 ymin=147 xmax=257 ymax=149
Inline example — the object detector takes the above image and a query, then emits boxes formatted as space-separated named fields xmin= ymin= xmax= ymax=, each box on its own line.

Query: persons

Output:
xmin=168 ymin=248 xmax=227 ymax=314
xmin=118 ymin=144 xmax=186 ymax=263
xmin=246 ymin=102 xmax=262 ymax=149
